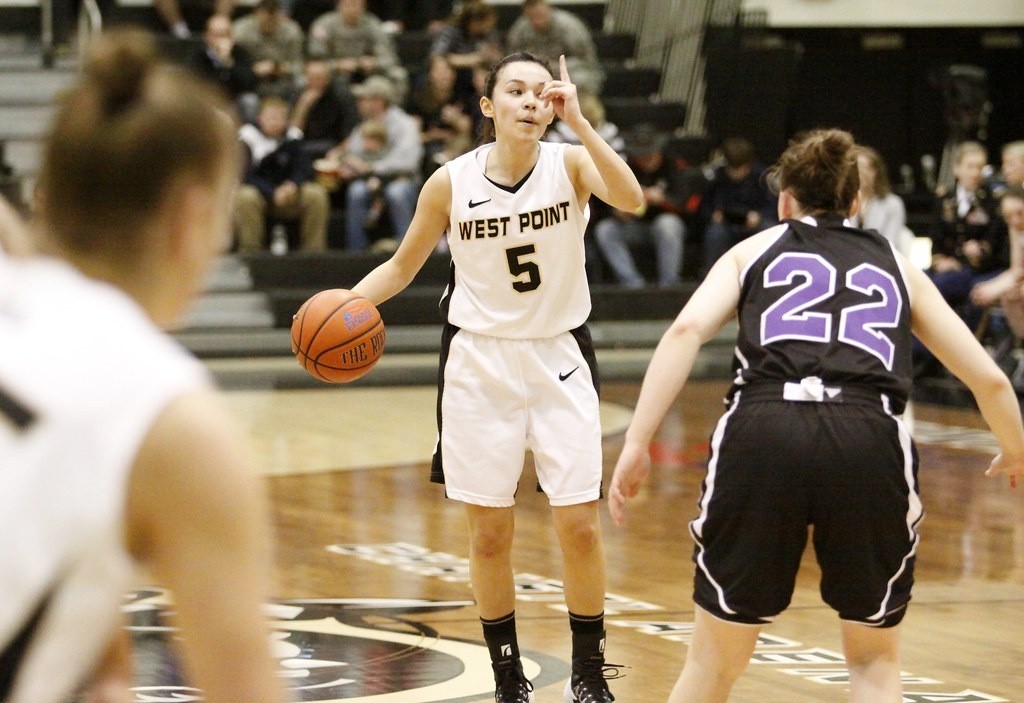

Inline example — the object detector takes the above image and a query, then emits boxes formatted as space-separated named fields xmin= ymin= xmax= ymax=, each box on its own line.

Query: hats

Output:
xmin=624 ymin=122 xmax=671 ymax=155
xmin=349 ymin=75 xmax=395 ymax=101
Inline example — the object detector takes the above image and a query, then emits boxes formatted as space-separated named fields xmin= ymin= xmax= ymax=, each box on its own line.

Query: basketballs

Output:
xmin=289 ymin=286 xmax=387 ymax=385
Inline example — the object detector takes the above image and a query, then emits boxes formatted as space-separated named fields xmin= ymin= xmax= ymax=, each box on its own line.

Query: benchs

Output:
xmin=62 ymin=0 xmax=721 ymax=329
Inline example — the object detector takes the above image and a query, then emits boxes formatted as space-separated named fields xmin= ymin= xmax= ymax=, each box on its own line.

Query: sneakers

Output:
xmin=564 ymin=653 xmax=625 ymax=703
xmin=492 ymin=659 xmax=533 ymax=703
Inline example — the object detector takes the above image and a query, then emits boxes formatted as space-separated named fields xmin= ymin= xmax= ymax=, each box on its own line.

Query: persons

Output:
xmin=591 ymin=123 xmax=686 ymax=291
xmin=0 ymin=28 xmax=300 ymax=703
xmin=350 ymin=52 xmax=644 ymax=703
xmin=193 ymin=0 xmax=1024 ymax=258
xmin=608 ymin=129 xmax=1024 ymax=703
xmin=849 ymin=145 xmax=915 ymax=259
xmin=924 ymin=137 xmax=1024 ymax=332
xmin=701 ymin=139 xmax=776 ymax=274
